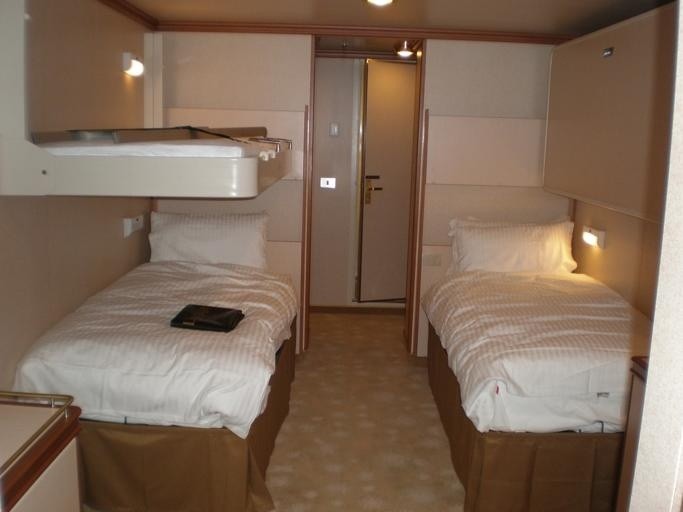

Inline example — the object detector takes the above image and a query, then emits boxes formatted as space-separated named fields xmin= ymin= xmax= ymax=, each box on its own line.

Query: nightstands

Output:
xmin=2 ymin=387 xmax=80 ymax=512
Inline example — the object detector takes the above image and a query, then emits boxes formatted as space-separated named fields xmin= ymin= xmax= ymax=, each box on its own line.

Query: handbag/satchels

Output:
xmin=170 ymin=304 xmax=244 ymax=332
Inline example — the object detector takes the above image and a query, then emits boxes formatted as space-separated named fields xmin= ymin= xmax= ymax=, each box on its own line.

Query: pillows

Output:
xmin=148 ymin=208 xmax=273 ymax=269
xmin=441 ymin=211 xmax=579 ymax=278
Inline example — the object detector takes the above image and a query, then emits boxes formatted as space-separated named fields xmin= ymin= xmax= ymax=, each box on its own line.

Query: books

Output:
xmin=630 ymin=355 xmax=648 ymax=383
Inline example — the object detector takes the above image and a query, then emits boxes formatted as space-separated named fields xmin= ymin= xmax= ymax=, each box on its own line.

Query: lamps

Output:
xmin=119 ymin=49 xmax=146 ymax=80
xmin=580 ymin=223 xmax=606 ymax=252
xmin=394 ymin=39 xmax=415 ymax=59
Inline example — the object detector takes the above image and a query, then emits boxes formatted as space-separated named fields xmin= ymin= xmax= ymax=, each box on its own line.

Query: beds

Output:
xmin=15 ymin=255 xmax=302 ymax=512
xmin=421 ymin=253 xmax=653 ymax=512
xmin=23 ymin=122 xmax=291 ymax=201
